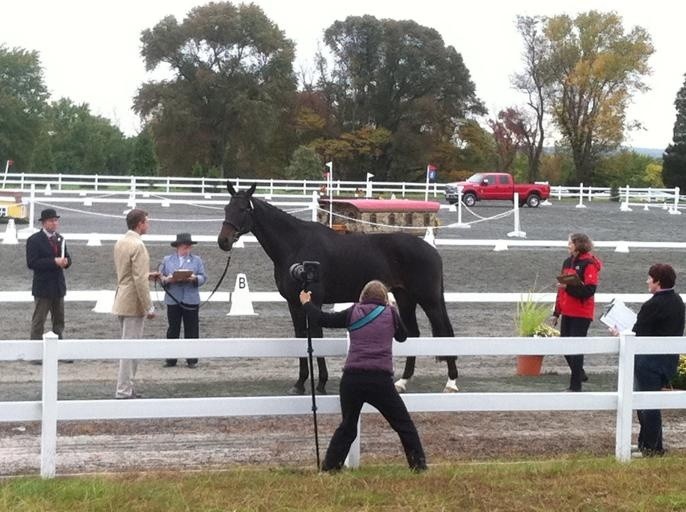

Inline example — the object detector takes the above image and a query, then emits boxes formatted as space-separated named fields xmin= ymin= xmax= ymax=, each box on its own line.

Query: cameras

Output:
xmin=287 ymin=261 xmax=320 ymax=298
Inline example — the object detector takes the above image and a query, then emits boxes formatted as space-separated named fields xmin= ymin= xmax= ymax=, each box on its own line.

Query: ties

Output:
xmin=51 ymin=236 xmax=58 ymax=256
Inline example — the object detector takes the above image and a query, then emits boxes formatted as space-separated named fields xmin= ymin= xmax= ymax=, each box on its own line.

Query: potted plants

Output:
xmin=514 ymin=270 xmax=561 ymax=376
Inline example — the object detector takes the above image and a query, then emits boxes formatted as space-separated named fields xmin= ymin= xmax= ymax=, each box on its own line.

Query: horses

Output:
xmin=218 ymin=180 xmax=459 ymax=395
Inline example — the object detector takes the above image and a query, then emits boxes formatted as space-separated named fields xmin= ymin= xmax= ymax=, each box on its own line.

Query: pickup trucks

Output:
xmin=444 ymin=172 xmax=550 ymax=208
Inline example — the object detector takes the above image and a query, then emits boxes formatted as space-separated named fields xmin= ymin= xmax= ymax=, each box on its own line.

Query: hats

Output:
xmin=38 ymin=209 xmax=60 ymax=221
xmin=171 ymin=233 xmax=197 ymax=247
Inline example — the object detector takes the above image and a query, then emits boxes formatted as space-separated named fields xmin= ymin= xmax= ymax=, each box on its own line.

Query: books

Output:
xmin=168 ymin=270 xmax=192 ymax=283
xmin=597 ymin=298 xmax=640 ymax=337
xmin=555 ymin=274 xmax=584 ymax=290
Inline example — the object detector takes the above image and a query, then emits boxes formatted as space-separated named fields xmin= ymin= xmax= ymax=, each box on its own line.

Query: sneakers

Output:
xmin=632 ymin=452 xmax=660 ymax=458
xmin=631 ymin=445 xmax=641 ymax=451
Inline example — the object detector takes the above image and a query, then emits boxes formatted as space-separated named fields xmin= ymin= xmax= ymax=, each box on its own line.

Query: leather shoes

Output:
xmin=163 ymin=362 xmax=173 ymax=366
xmin=189 ymin=363 xmax=197 ymax=368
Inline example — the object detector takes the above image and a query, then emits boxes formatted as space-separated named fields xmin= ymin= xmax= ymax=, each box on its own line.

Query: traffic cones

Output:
xmin=225 ymin=273 xmax=258 ymax=317
xmin=42 ymin=182 xmax=52 ymax=195
xmin=0 ymin=219 xmax=20 ymax=244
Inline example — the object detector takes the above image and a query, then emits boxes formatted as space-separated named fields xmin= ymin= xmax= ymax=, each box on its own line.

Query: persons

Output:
xmin=27 ymin=209 xmax=73 ymax=365
xmin=299 ymin=279 xmax=429 ymax=475
xmin=158 ymin=232 xmax=207 ymax=369
xmin=608 ymin=262 xmax=685 ymax=458
xmin=550 ymin=233 xmax=602 ymax=391
xmin=115 ymin=209 xmax=162 ymax=400
